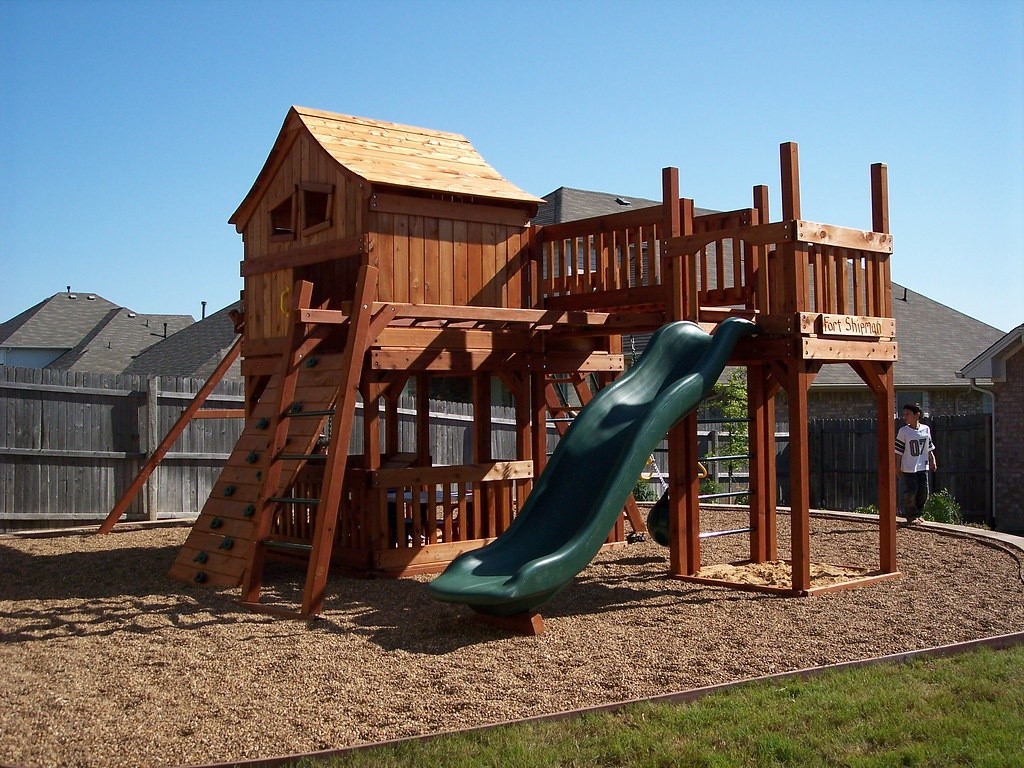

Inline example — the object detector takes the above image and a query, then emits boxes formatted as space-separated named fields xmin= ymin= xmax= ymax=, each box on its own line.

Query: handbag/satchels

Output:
xmin=646 ymin=488 xmax=669 ymax=547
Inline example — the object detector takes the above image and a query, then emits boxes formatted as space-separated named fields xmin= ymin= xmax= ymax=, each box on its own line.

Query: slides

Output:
xmin=425 ymin=316 xmax=757 ymax=616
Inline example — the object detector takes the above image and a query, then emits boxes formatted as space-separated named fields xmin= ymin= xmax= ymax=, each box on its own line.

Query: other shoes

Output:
xmin=918 ymin=517 xmax=924 ymax=523
xmin=907 ymin=519 xmax=921 ymax=525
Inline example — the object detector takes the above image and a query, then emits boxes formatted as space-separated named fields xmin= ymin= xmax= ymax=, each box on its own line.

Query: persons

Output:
xmin=895 ymin=403 xmax=937 ymax=525
